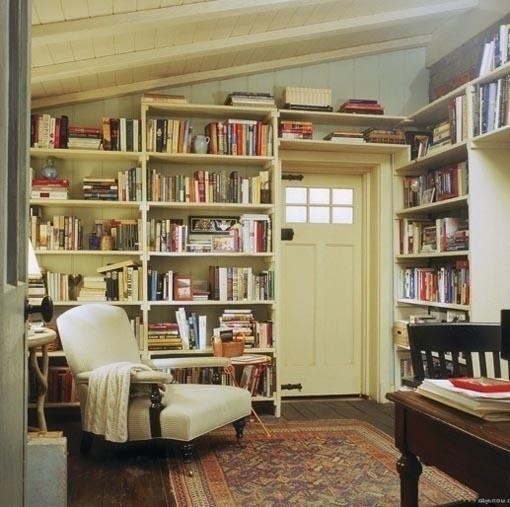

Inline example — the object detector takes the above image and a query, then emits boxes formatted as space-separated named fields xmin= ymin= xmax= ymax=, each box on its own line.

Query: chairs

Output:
xmin=55 ymin=303 xmax=251 ymax=476
xmin=401 ymin=323 xmax=509 ymax=387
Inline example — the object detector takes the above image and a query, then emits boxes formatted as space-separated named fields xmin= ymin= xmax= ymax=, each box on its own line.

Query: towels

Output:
xmin=82 ymin=362 xmax=164 ymax=443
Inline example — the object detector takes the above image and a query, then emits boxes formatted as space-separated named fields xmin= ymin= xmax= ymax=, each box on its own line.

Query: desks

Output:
xmin=385 ymin=382 xmax=510 ymax=507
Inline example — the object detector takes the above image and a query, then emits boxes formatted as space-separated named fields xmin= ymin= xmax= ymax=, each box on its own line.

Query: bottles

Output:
xmin=40 ymin=155 xmax=56 ymax=179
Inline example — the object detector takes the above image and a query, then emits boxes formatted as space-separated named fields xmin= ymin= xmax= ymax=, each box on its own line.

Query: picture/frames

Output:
xmin=189 ymin=215 xmax=240 ymax=235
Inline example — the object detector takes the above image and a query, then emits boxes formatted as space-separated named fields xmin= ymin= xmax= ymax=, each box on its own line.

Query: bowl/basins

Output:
xmin=222 ymin=341 xmax=244 ymax=357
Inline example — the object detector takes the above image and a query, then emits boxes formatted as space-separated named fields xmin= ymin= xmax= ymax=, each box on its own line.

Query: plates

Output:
xmin=245 ymin=344 xmax=253 ymax=347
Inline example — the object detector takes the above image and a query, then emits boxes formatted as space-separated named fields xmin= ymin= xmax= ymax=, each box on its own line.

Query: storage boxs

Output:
xmin=393 ymin=320 xmax=409 ymax=345
xmin=282 ymin=86 xmax=331 ymax=106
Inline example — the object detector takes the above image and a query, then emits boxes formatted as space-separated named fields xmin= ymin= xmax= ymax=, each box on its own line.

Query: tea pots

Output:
xmin=192 ymin=131 xmax=210 ymax=153
xmin=210 ymin=335 xmax=224 ymax=357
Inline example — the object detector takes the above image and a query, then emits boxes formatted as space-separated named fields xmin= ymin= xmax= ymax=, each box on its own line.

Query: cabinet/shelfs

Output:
xmin=277 ymin=62 xmax=510 ymax=389
xmin=27 ymin=103 xmax=282 ymax=418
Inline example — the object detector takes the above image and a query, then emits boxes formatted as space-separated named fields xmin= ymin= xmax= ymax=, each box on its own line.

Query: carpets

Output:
xmin=161 ymin=418 xmax=480 ymax=507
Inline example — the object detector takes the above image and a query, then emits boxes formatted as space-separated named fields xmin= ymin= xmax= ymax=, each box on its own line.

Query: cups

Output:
xmin=88 ymin=222 xmax=113 ymax=251
